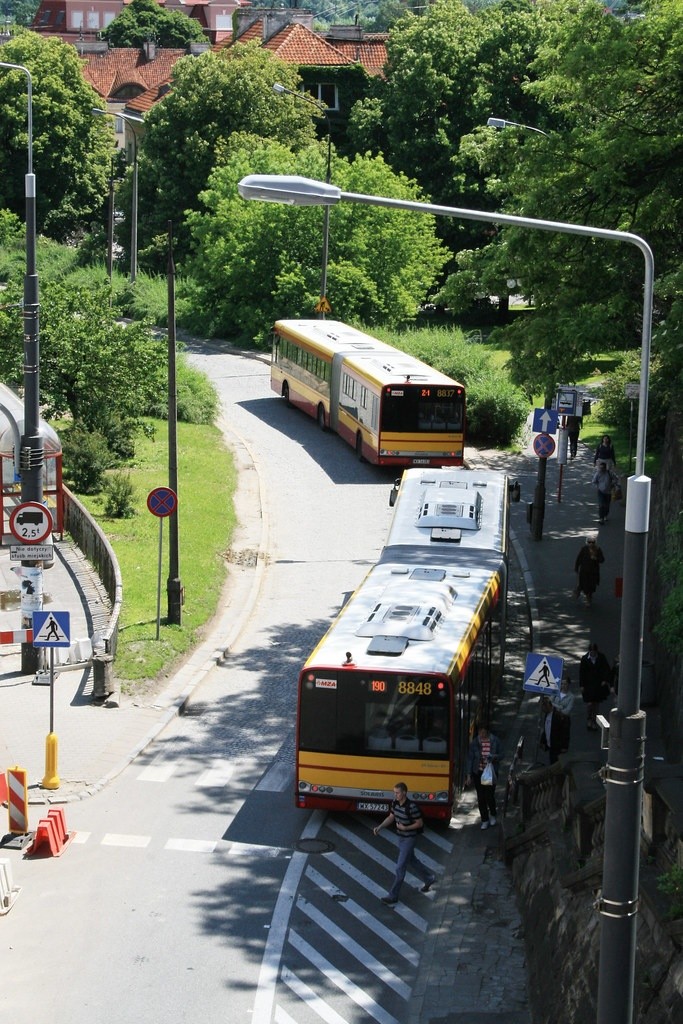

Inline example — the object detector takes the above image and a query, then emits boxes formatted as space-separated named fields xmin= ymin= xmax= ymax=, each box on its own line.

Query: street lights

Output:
xmin=236 ymin=174 xmax=657 ymax=1024
xmin=95 ymin=107 xmax=138 ymax=286
xmin=272 ymin=82 xmax=331 ymax=320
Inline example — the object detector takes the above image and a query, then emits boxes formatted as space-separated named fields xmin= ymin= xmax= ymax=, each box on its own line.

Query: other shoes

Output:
xmin=592 ymin=722 xmax=598 ymax=730
xmin=604 ymin=515 xmax=607 ymax=520
xmin=598 ymin=519 xmax=604 ymax=524
xmin=587 ymin=718 xmax=592 ymax=727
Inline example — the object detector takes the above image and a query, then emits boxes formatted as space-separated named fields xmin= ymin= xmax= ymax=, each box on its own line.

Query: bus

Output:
xmin=293 ymin=467 xmax=522 ymax=823
xmin=269 ymin=319 xmax=472 ymax=468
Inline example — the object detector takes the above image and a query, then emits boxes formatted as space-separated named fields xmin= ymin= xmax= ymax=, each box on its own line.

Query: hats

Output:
xmin=587 ymin=643 xmax=599 ymax=649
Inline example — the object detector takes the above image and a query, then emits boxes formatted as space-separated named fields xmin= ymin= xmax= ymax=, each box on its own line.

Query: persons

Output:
xmin=550 ymin=675 xmax=575 ymax=715
xmin=566 ymin=416 xmax=583 ymax=459
xmin=575 ymin=536 xmax=604 ymax=607
xmin=465 ymin=722 xmax=504 ymax=829
xmin=593 ymin=435 xmax=616 ymax=470
xmin=370 ymin=713 xmax=402 ymax=742
xmin=374 ymin=782 xmax=435 ymax=904
xmin=592 ymin=463 xmax=620 ymax=524
xmin=579 ymin=643 xmax=619 ymax=731
xmin=539 ymin=697 xmax=570 ymax=767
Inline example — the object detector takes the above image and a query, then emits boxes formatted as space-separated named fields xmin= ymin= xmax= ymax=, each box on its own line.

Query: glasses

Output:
xmin=586 ymin=540 xmax=596 ymax=544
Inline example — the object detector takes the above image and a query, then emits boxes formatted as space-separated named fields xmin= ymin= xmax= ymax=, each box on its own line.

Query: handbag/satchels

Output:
xmin=481 ymin=761 xmax=499 ymax=786
xmin=610 ymin=485 xmax=622 ymax=501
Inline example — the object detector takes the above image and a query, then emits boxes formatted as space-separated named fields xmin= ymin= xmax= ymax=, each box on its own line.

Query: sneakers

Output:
xmin=419 ymin=875 xmax=439 ymax=893
xmin=480 ymin=821 xmax=489 ymax=830
xmin=490 ymin=815 xmax=497 ymax=825
xmin=381 ymin=897 xmax=398 ymax=907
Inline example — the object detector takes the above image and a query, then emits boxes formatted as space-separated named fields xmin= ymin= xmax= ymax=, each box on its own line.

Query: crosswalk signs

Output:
xmin=523 ymin=650 xmax=565 ymax=696
xmin=32 ymin=610 xmax=71 ymax=648
